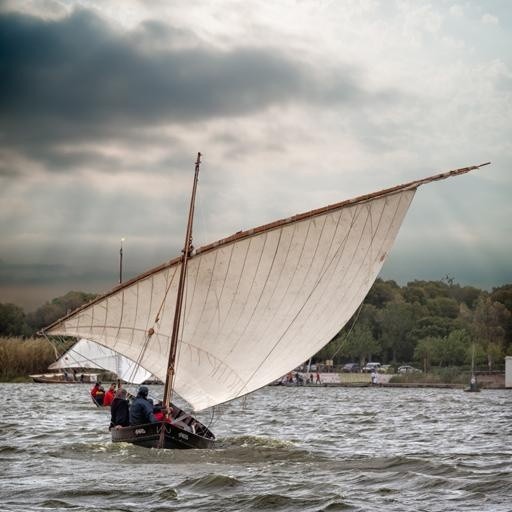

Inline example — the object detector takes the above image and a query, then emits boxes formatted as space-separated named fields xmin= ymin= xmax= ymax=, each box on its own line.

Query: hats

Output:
xmin=140 ymin=387 xmax=148 ymax=395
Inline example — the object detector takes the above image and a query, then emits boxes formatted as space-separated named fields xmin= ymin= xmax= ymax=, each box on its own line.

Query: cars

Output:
xmin=296 ymin=362 xmax=423 ymax=374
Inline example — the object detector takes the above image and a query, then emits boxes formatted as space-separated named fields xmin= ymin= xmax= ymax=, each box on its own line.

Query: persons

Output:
xmin=64 ymin=370 xmax=84 ymax=381
xmin=92 ymin=382 xmax=171 ymax=430
xmin=287 ymin=372 xmax=321 ymax=385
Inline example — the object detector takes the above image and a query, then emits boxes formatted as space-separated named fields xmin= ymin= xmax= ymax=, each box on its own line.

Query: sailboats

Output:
xmin=35 ymin=152 xmax=491 ymax=449
xmin=28 ymin=236 xmax=165 ymax=386
